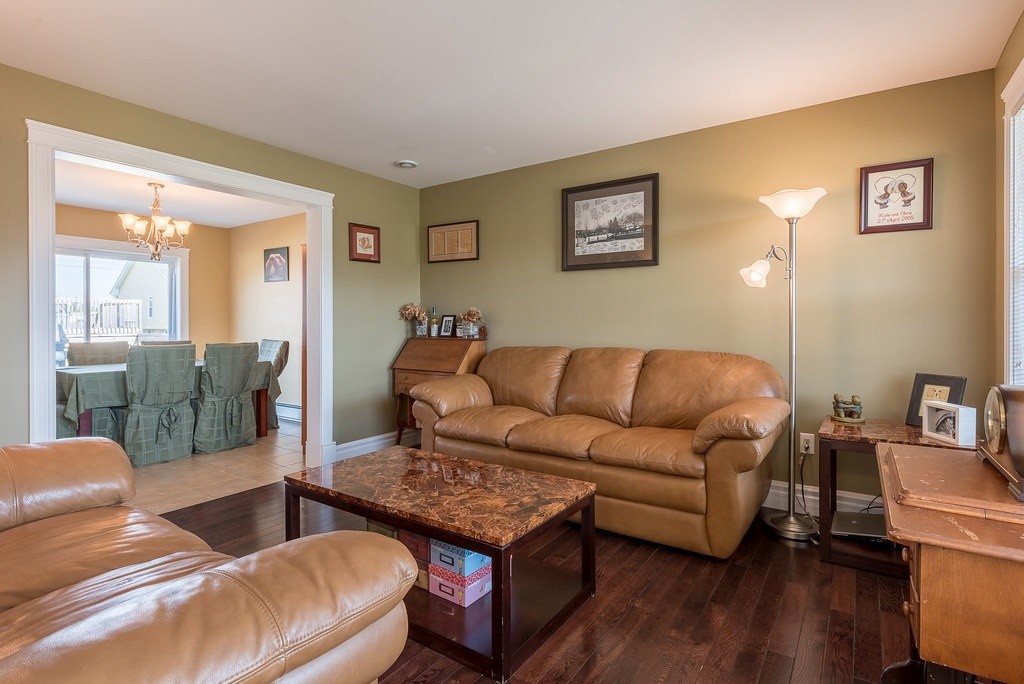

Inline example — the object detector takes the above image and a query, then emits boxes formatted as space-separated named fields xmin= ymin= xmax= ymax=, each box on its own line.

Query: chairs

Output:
xmin=55 ymin=339 xmax=289 ymax=469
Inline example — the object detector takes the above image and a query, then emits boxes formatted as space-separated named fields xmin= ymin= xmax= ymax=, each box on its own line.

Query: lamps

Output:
xmin=739 ymin=187 xmax=827 ymax=542
xmin=118 ymin=182 xmax=191 ymax=261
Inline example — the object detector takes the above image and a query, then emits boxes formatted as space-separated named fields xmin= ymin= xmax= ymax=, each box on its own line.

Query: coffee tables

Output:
xmin=285 ymin=444 xmax=598 ymax=684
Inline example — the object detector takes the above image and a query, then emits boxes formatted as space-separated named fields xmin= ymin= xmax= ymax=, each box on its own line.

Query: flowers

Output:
xmin=462 ymin=306 xmax=482 ymax=324
xmin=400 ymin=302 xmax=428 ymax=321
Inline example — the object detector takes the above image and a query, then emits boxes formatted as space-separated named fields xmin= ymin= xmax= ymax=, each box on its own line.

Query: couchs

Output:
xmin=0 ymin=434 xmax=418 ymax=684
xmin=413 ymin=346 xmax=791 ymax=564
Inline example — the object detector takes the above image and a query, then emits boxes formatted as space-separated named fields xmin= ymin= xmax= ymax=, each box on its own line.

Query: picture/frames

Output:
xmin=561 ymin=172 xmax=660 ymax=273
xmin=263 ymin=246 xmax=289 ymax=283
xmin=440 ymin=315 xmax=456 ymax=337
xmin=922 ymin=400 xmax=976 ymax=446
xmin=904 ymin=373 xmax=967 ymax=427
xmin=348 ymin=222 xmax=381 ymax=264
xmin=858 ymin=157 xmax=935 ymax=235
xmin=427 ymin=219 xmax=479 ymax=263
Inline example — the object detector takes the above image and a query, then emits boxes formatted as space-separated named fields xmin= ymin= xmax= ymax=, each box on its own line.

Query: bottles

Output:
xmin=430 ymin=306 xmax=439 ymax=336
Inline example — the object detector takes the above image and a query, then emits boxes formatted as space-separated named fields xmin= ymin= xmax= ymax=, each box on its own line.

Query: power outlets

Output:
xmin=799 ymin=433 xmax=815 ymax=455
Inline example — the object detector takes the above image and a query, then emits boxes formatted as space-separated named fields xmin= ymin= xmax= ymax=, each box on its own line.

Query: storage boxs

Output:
xmin=363 ymin=516 xmax=513 ymax=608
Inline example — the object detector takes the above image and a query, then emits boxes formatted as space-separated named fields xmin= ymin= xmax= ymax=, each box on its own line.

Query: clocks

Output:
xmin=975 ymin=383 xmax=1024 ymax=503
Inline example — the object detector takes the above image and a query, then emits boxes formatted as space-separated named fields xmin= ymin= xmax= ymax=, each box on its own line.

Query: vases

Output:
xmin=415 ymin=320 xmax=427 ymax=337
xmin=462 ymin=320 xmax=479 ymax=339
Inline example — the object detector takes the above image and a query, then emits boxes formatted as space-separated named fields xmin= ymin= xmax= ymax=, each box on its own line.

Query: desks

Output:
xmin=818 ymin=414 xmax=985 ymax=581
xmin=56 ymin=359 xmax=281 ymax=444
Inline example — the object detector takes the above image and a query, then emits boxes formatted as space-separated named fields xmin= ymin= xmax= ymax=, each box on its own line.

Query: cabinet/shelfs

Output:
xmin=390 ymin=336 xmax=488 ymax=446
xmin=874 ymin=442 xmax=1024 ymax=684
